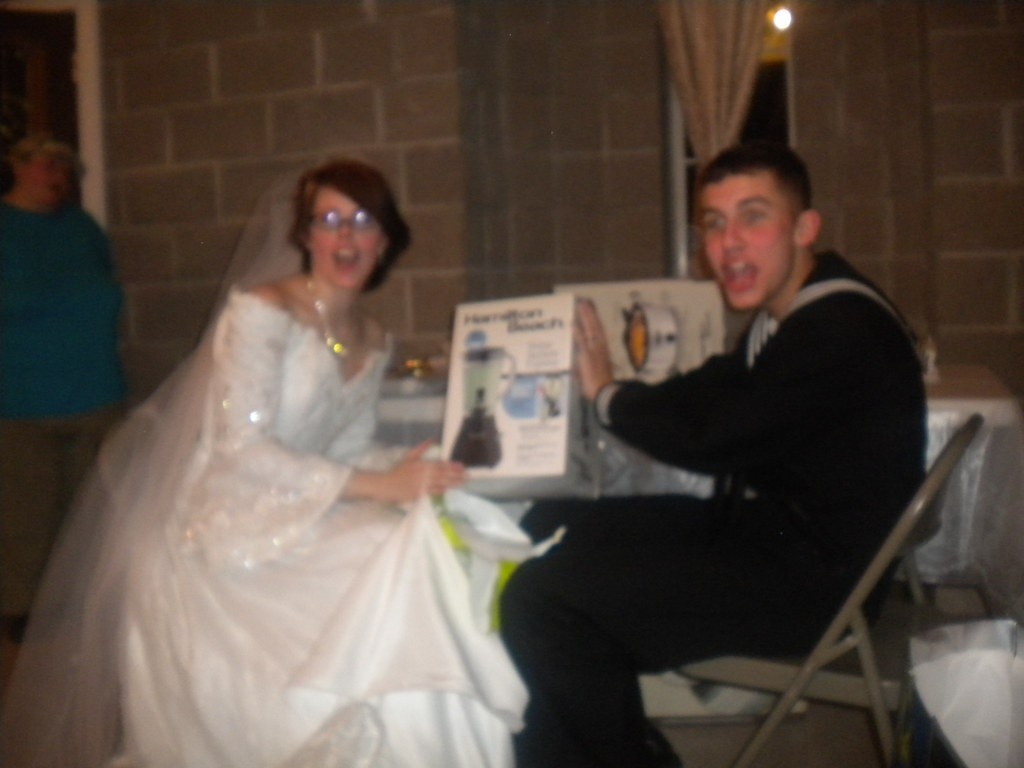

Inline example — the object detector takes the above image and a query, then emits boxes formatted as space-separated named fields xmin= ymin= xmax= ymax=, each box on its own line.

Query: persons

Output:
xmin=0 ymin=112 xmax=135 ymax=711
xmin=4 ymin=155 xmax=531 ymax=766
xmin=490 ymin=137 xmax=925 ymax=763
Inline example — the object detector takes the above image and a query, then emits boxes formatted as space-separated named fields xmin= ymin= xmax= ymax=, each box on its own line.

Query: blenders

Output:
xmin=450 ymin=348 xmax=517 ymax=469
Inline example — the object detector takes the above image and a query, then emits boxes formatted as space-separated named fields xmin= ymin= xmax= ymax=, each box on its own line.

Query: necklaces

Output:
xmin=303 ymin=277 xmax=368 ymax=357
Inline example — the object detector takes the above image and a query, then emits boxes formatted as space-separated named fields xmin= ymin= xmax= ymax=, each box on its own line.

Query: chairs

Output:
xmin=660 ymin=409 xmax=984 ymax=767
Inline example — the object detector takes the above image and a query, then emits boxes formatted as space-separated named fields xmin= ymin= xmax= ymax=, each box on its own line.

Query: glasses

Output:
xmin=307 ymin=207 xmax=376 ymax=232
